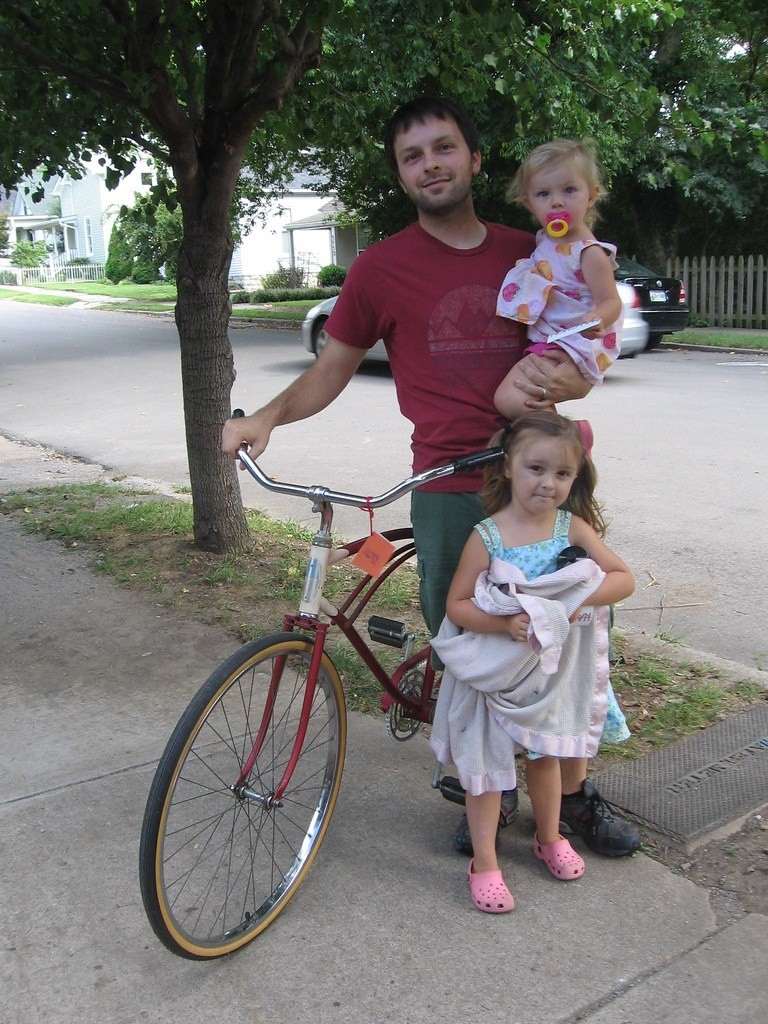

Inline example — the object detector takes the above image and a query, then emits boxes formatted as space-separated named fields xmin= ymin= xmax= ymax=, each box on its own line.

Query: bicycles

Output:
xmin=136 ymin=408 xmax=616 ymax=962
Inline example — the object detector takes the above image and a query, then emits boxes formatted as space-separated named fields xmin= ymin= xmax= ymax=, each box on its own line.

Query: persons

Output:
xmin=222 ymin=97 xmax=641 ymax=859
xmin=494 ymin=139 xmax=623 ymax=460
xmin=446 ymin=412 xmax=635 ymax=913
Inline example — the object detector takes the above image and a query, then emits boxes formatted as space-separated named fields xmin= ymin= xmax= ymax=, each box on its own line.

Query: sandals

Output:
xmin=464 ymin=856 xmax=514 ymax=911
xmin=532 ymin=830 xmax=585 ymax=879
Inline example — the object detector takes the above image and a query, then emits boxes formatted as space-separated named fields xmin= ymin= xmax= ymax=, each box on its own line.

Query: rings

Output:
xmin=542 ymin=389 xmax=546 ymax=397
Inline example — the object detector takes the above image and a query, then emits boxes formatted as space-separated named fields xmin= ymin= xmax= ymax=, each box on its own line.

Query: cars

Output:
xmin=301 ymin=281 xmax=649 ymax=365
xmin=609 ymin=255 xmax=689 ymax=352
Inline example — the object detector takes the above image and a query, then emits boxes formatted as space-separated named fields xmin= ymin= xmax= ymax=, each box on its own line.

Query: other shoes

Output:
xmin=574 ymin=418 xmax=593 ymax=466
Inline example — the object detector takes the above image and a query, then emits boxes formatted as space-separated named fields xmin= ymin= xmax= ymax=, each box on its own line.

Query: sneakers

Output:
xmin=453 ymin=785 xmax=521 ymax=856
xmin=562 ymin=776 xmax=640 ymax=859
